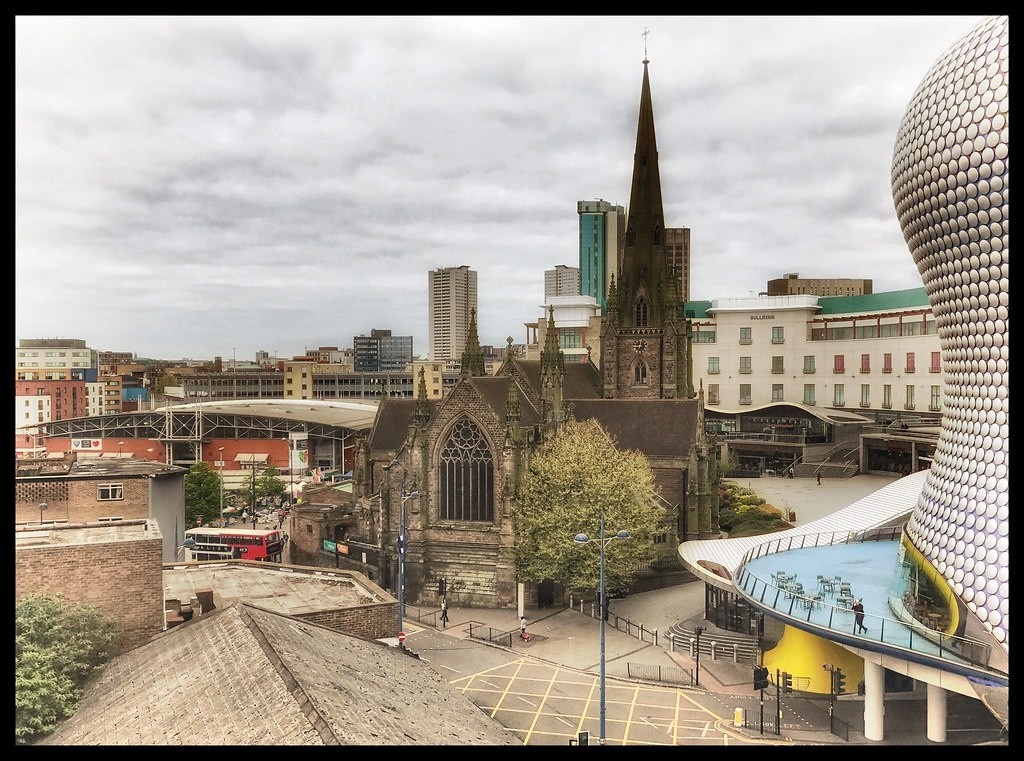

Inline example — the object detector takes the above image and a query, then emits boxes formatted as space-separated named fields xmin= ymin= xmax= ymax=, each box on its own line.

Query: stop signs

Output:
xmin=398 ymin=632 xmax=406 ymax=642
xmin=196 ymin=517 xmax=201 ymax=526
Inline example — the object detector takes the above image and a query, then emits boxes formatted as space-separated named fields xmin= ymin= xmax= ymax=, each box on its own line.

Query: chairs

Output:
xmin=773 ymin=571 xmax=864 ymax=613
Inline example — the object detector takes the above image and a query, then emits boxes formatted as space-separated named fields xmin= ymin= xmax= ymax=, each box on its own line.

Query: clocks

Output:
xmin=632 ymin=339 xmax=647 ymax=353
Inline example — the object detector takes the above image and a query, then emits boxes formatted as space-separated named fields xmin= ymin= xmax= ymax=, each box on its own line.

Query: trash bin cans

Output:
xmin=789 ymin=512 xmax=796 ymax=522
xmin=733 ymin=707 xmax=743 ymax=727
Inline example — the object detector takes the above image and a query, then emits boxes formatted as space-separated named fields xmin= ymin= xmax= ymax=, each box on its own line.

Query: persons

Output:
xmin=241 ymin=510 xmax=247 ymax=524
xmin=279 ymin=514 xmax=284 ymax=528
xmin=817 ymin=471 xmax=821 ymax=485
xmin=281 ymin=508 xmax=286 ymax=519
xmin=520 ymin=617 xmax=527 ymax=637
xmin=787 ymin=467 xmax=793 ymax=478
xmin=851 ymin=599 xmax=868 ymax=634
xmin=438 ymin=603 xmax=449 ymax=622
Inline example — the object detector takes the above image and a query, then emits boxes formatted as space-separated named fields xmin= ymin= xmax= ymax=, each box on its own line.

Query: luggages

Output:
xmin=522 ymin=630 xmax=530 ymax=642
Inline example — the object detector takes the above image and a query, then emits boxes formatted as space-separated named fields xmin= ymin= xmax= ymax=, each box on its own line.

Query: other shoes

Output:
xmin=865 ymin=628 xmax=867 ymax=634
xmin=858 ymin=631 xmax=861 ymax=634
xmin=446 ymin=620 xmax=448 ymax=622
xmin=439 ymin=617 xmax=442 ymax=620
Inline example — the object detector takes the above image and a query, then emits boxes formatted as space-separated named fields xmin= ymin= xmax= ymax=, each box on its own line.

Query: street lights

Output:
xmin=573 ymin=514 xmax=631 ymax=747
xmin=397 ymin=490 xmax=421 ymax=646
xmin=217 ymin=446 xmax=224 ymax=518
xmin=118 ymin=441 xmax=124 ymax=457
xmin=38 ymin=503 xmax=48 ymax=525
xmin=282 ymin=436 xmax=294 ymax=506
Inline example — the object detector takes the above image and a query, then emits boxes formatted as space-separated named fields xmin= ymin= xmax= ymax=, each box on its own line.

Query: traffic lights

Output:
xmin=834 ymin=669 xmax=846 ymax=694
xmin=781 ymin=672 xmax=792 ymax=694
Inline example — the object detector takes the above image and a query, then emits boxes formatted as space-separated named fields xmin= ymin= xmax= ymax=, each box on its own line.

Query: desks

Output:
xmin=803 ymin=594 xmax=813 ymax=608
xmin=778 ymin=575 xmax=788 ymax=579
xmin=840 ymin=586 xmax=852 ymax=598
xmin=785 ymin=583 xmax=796 ymax=598
xmin=838 ymin=597 xmax=851 ymax=612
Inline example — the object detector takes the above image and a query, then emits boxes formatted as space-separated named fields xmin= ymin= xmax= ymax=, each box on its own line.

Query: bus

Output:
xmin=185 ymin=526 xmax=286 ymax=561
